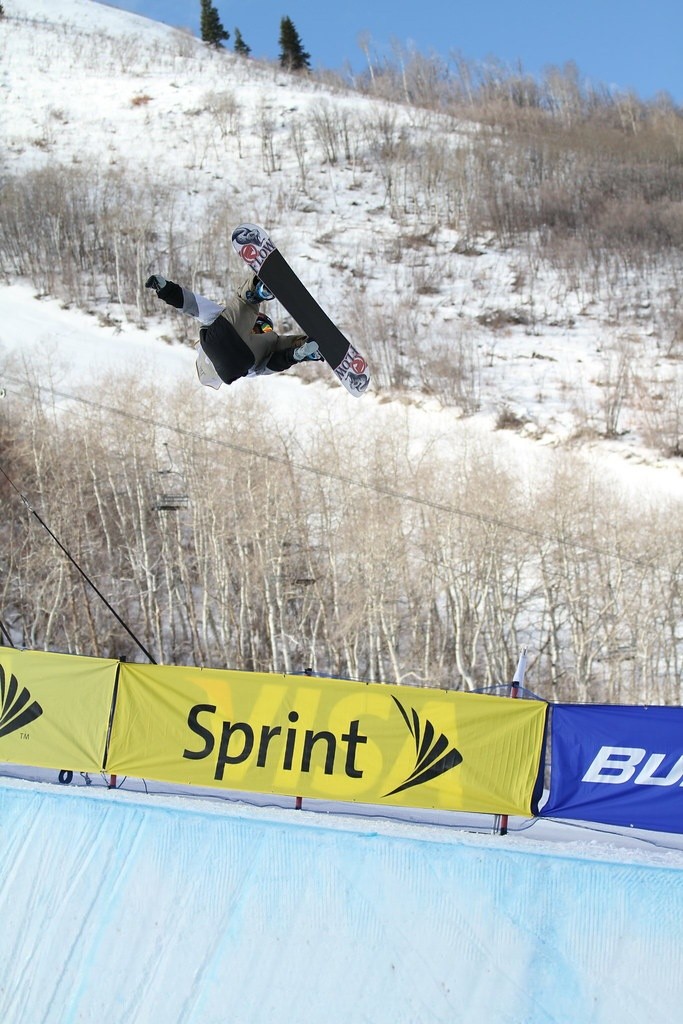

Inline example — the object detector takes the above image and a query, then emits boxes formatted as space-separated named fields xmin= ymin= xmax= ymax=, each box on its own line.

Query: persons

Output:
xmin=145 ymin=274 xmax=324 ymax=390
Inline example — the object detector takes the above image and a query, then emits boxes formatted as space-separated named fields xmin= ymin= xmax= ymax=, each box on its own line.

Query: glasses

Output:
xmin=260 ymin=323 xmax=273 ymax=334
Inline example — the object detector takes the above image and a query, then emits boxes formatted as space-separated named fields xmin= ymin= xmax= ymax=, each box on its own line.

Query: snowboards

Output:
xmin=230 ymin=222 xmax=373 ymax=397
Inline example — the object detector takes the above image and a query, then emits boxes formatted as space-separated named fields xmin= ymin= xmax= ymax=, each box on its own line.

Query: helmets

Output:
xmin=253 ymin=313 xmax=273 ymax=334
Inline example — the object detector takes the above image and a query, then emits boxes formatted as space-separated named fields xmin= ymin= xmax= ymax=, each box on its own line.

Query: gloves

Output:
xmin=145 ymin=275 xmax=166 ymax=291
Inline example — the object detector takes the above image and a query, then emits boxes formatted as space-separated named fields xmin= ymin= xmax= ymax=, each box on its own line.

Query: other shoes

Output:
xmin=254 ymin=281 xmax=275 ymax=299
xmin=304 ymin=342 xmax=322 ymax=360
